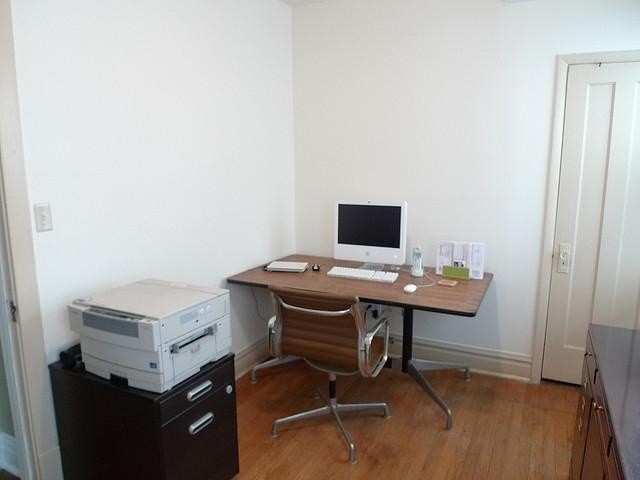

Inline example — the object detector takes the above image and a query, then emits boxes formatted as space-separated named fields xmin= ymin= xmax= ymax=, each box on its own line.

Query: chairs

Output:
xmin=251 ymin=284 xmax=392 ymax=465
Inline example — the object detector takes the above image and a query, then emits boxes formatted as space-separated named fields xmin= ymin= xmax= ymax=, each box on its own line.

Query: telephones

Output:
xmin=410 ymin=245 xmax=423 ymax=277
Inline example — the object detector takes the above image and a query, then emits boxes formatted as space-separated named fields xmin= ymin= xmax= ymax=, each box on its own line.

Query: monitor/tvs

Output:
xmin=333 ymin=201 xmax=408 ymax=271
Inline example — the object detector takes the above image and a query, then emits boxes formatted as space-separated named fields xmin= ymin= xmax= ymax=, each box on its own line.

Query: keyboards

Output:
xmin=327 ymin=266 xmax=399 ymax=284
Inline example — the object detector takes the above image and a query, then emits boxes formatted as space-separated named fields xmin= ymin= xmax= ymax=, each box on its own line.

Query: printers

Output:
xmin=68 ymin=278 xmax=232 ymax=394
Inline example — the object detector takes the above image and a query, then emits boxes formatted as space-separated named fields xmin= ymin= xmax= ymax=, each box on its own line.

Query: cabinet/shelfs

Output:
xmin=569 ymin=323 xmax=640 ymax=480
xmin=48 ymin=357 xmax=239 ymax=479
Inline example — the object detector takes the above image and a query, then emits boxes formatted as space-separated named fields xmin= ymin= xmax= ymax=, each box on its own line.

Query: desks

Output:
xmin=227 ymin=254 xmax=494 ymax=431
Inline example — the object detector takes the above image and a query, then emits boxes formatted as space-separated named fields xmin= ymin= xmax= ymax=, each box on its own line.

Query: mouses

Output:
xmin=403 ymin=284 xmax=417 ymax=293
